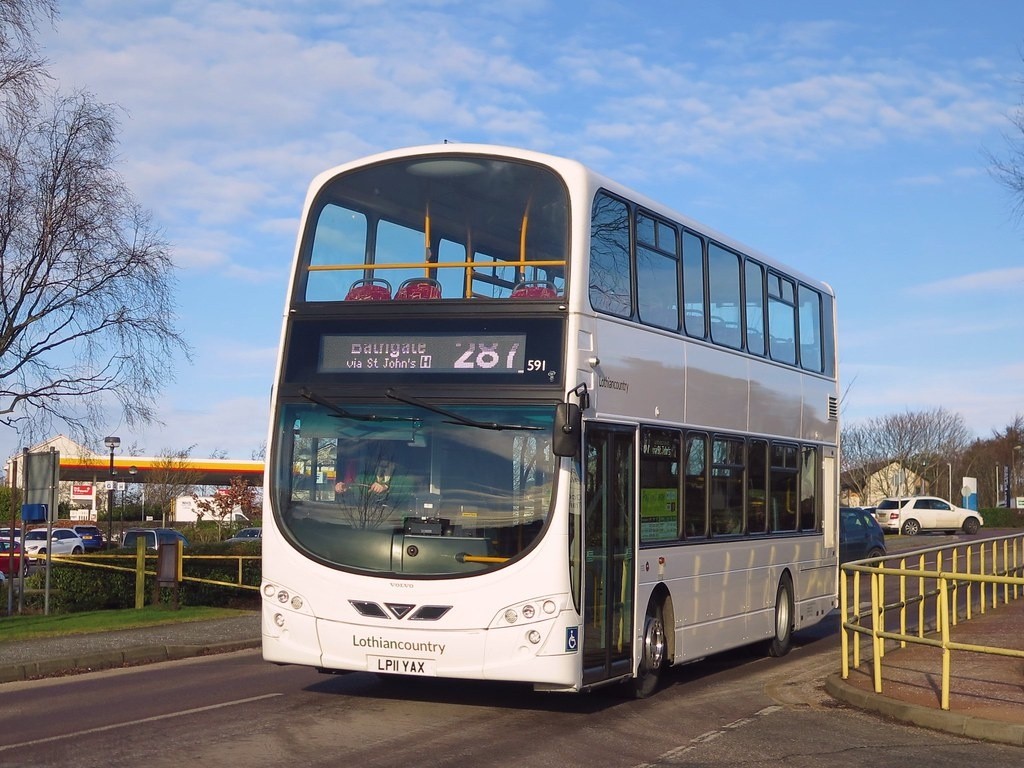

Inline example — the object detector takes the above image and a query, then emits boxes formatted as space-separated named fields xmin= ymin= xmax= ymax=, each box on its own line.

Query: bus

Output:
xmin=260 ymin=140 xmax=842 ymax=698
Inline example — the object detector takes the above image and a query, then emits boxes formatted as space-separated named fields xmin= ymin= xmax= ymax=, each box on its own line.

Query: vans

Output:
xmin=121 ymin=527 xmax=190 ymax=551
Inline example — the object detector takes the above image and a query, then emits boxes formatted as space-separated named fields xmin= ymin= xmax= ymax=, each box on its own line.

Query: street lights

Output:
xmin=946 ymin=463 xmax=951 ymax=504
xmin=1012 ymin=445 xmax=1021 ymax=508
xmin=105 ymin=436 xmax=139 ymax=553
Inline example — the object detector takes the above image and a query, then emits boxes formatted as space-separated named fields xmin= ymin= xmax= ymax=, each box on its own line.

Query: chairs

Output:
xmin=344 ymin=279 xmax=392 ymax=301
xmin=394 ymin=278 xmax=442 ymax=299
xmin=509 ymin=281 xmax=558 ymax=300
xmin=665 ymin=303 xmax=792 ymax=363
xmin=847 ymin=516 xmax=859 ymax=530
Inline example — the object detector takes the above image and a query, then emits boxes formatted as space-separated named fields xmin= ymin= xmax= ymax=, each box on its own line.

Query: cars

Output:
xmin=839 ymin=507 xmax=887 ymax=574
xmin=875 ymin=496 xmax=984 ymax=535
xmin=861 ymin=506 xmax=877 ymax=519
xmin=16 ymin=528 xmax=85 ymax=565
xmin=223 ymin=527 xmax=262 ymax=542
xmin=0 ymin=527 xmax=21 ymax=544
xmin=73 ymin=524 xmax=104 ymax=551
xmin=0 ymin=538 xmax=29 ymax=578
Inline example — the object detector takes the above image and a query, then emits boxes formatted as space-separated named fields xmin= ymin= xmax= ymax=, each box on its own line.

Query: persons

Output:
xmin=335 ymin=440 xmax=411 ymax=506
